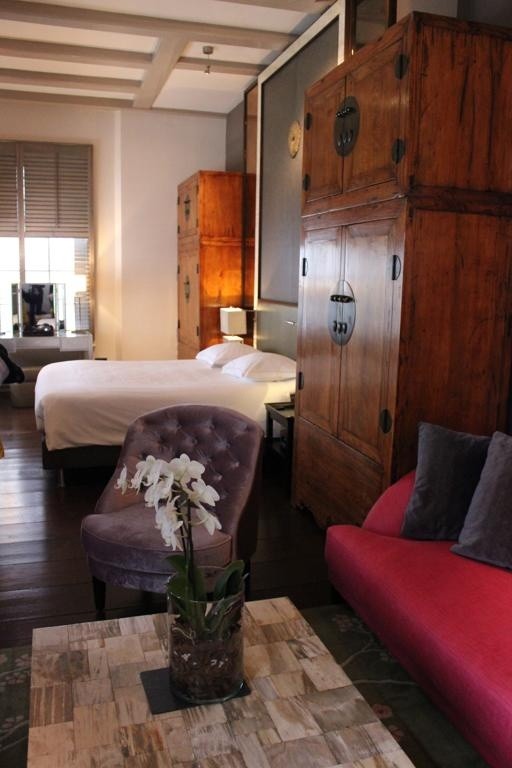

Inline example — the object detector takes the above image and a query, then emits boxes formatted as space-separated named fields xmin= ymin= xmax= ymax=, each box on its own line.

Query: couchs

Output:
xmin=324 ymin=469 xmax=512 ymax=767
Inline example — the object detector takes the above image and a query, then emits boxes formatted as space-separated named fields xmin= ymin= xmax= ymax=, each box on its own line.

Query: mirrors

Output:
xmin=12 ymin=282 xmax=56 ymax=338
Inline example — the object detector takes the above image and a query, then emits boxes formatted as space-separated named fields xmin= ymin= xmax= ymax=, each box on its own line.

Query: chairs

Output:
xmin=79 ymin=402 xmax=264 ymax=614
xmin=9 ymin=347 xmax=61 ymax=409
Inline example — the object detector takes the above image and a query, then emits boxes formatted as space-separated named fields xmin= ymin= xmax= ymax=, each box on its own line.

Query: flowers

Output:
xmin=116 ymin=455 xmax=250 ymax=644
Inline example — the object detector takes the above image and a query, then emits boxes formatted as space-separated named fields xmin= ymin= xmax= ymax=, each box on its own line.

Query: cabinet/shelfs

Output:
xmin=176 ymin=169 xmax=255 ymax=359
xmin=293 ymin=8 xmax=512 ymax=536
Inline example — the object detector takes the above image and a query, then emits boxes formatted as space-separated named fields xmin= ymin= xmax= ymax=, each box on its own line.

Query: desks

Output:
xmin=264 ymin=402 xmax=294 ymax=462
xmin=0 ymin=329 xmax=93 ymax=362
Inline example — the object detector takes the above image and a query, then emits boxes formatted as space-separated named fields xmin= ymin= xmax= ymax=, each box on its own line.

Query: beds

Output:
xmin=34 ymin=359 xmax=294 ymax=489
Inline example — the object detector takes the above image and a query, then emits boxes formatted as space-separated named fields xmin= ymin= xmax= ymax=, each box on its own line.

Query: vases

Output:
xmin=165 ymin=564 xmax=248 ymax=704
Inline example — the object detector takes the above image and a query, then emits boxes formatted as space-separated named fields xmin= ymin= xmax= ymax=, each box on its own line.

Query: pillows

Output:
xmin=221 ymin=352 xmax=297 ymax=383
xmin=401 ymin=419 xmax=491 ymax=543
xmin=450 ymin=430 xmax=512 ymax=572
xmin=195 ymin=342 xmax=261 ymax=369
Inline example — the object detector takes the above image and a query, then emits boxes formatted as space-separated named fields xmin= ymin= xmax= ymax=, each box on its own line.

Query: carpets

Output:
xmin=0 ymin=600 xmax=486 ymax=767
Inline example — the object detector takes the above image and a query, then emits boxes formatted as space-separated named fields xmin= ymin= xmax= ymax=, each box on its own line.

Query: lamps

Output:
xmin=220 ymin=307 xmax=246 ymax=345
